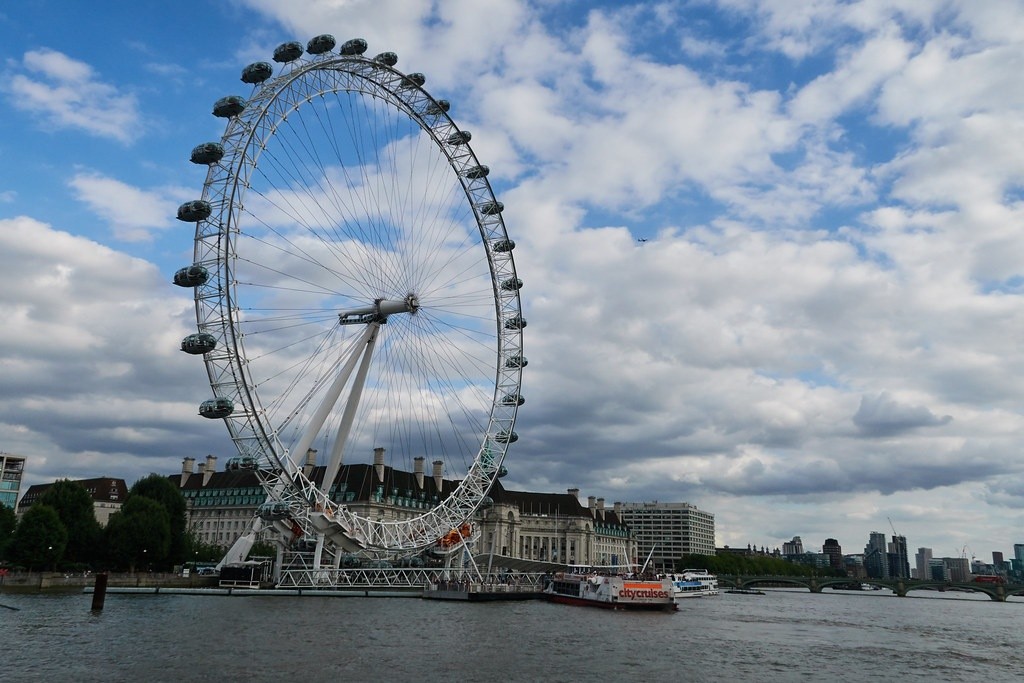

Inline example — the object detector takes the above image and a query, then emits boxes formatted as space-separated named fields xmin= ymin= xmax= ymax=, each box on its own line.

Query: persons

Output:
xmin=62 ymin=561 xmax=677 ymax=589
xmin=713 ymin=573 xmax=1024 ymax=585
xmin=0 ymin=566 xmax=7 ymax=583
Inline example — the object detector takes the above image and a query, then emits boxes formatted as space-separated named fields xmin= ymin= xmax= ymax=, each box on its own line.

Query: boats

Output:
xmin=659 ymin=568 xmax=719 ymax=598
xmin=540 ymin=540 xmax=681 ymax=611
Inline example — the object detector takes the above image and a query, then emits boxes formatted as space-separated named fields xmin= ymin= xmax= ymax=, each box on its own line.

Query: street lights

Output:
xmin=190 ymin=551 xmax=199 ymax=574
